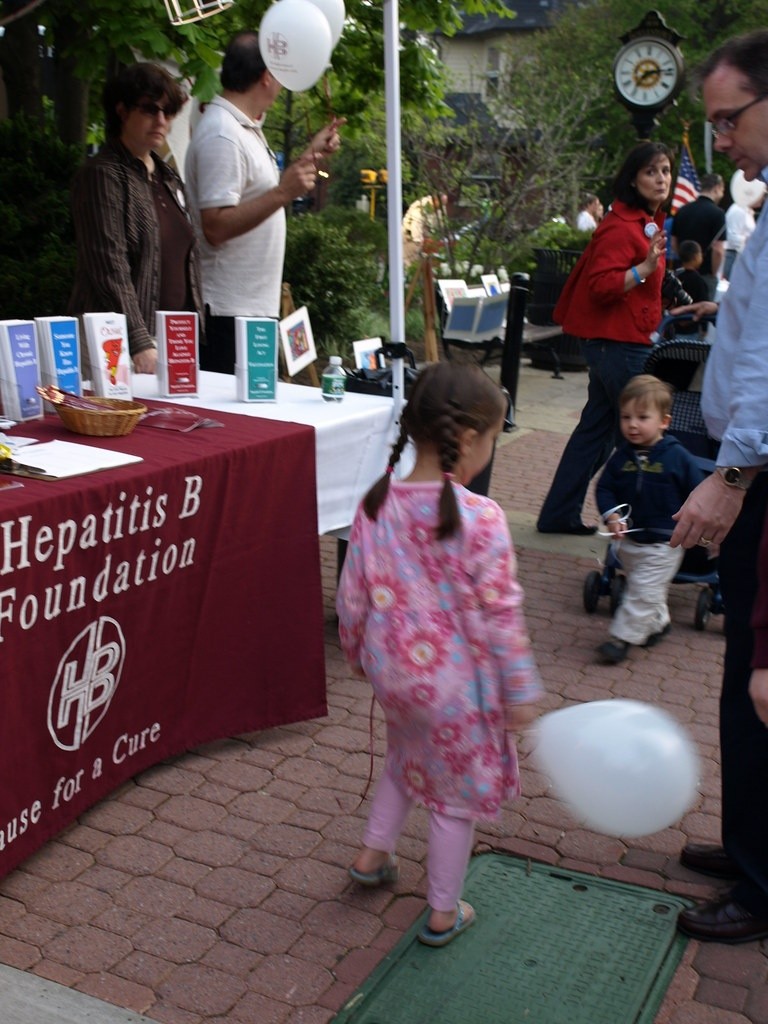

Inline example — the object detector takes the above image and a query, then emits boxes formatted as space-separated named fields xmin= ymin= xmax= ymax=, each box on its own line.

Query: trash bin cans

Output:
xmin=337 ymin=367 xmax=493 ymax=582
xmin=530 ymin=245 xmax=592 ymax=371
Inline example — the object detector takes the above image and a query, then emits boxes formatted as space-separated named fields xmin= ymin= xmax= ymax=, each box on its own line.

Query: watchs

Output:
xmin=716 ymin=466 xmax=751 ymax=490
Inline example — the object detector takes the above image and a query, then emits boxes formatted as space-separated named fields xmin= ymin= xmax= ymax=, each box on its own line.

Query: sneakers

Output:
xmin=641 ymin=623 xmax=672 ymax=649
xmin=597 ymin=637 xmax=627 ymax=665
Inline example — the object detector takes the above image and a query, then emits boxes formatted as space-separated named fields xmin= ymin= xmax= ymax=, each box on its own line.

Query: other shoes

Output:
xmin=573 ymin=522 xmax=598 ymax=535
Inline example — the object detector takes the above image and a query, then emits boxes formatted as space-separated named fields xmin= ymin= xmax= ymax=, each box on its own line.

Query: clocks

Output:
xmin=611 ymin=37 xmax=684 ymax=115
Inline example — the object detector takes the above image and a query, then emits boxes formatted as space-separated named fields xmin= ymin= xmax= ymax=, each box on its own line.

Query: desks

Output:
xmin=0 ymin=369 xmax=417 ymax=881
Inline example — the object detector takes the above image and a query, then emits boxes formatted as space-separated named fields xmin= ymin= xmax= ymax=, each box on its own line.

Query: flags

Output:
xmin=670 ymin=136 xmax=702 ymax=216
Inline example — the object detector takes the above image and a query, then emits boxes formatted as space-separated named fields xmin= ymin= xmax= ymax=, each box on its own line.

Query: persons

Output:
xmin=592 ymin=373 xmax=704 ymax=666
xmin=402 ymin=192 xmax=448 ymax=243
xmin=183 ymin=30 xmax=347 ymax=375
xmin=67 ymin=63 xmax=206 ymax=382
xmin=671 ymin=31 xmax=768 ymax=942
xmin=334 ymin=359 xmax=540 ymax=945
xmin=536 ymin=141 xmax=765 ymax=535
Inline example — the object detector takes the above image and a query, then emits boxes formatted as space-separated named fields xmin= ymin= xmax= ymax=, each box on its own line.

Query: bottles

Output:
xmin=321 ymin=356 xmax=347 ymax=403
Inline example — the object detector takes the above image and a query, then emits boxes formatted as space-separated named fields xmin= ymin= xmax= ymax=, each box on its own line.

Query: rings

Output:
xmin=700 ymin=537 xmax=712 ymax=546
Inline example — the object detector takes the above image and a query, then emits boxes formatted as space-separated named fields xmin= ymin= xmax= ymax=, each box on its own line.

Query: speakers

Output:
xmin=337 ymin=363 xmax=496 ymax=588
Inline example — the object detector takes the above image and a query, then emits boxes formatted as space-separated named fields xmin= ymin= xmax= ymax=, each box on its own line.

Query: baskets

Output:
xmin=53 ymin=396 xmax=148 ymax=437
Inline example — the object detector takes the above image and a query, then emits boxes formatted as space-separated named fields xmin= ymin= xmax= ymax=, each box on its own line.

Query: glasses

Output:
xmin=130 ymin=103 xmax=179 ymax=120
xmin=711 ymin=95 xmax=765 ymax=139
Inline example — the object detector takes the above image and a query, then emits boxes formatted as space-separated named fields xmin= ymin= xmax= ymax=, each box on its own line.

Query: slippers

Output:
xmin=351 ymin=852 xmax=401 ymax=883
xmin=419 ymin=900 xmax=474 ymax=947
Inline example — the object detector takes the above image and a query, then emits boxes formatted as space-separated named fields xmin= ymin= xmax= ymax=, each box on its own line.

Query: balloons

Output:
xmin=526 ymin=699 xmax=697 ymax=836
xmin=730 ymin=168 xmax=766 ymax=206
xmin=257 ymin=0 xmax=345 ymax=92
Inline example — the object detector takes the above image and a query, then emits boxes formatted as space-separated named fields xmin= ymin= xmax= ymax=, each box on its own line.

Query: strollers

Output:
xmin=581 ymin=310 xmax=727 ymax=636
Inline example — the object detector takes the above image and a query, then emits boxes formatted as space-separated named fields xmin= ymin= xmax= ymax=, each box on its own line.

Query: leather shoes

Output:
xmin=678 ymin=892 xmax=768 ymax=942
xmin=680 ymin=843 xmax=737 ymax=880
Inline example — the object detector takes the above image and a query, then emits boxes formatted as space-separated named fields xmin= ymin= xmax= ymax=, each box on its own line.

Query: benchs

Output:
xmin=434 ymin=280 xmax=566 ymax=380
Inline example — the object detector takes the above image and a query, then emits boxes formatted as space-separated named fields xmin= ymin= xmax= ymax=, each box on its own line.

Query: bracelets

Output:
xmin=631 ymin=267 xmax=641 ymax=284
